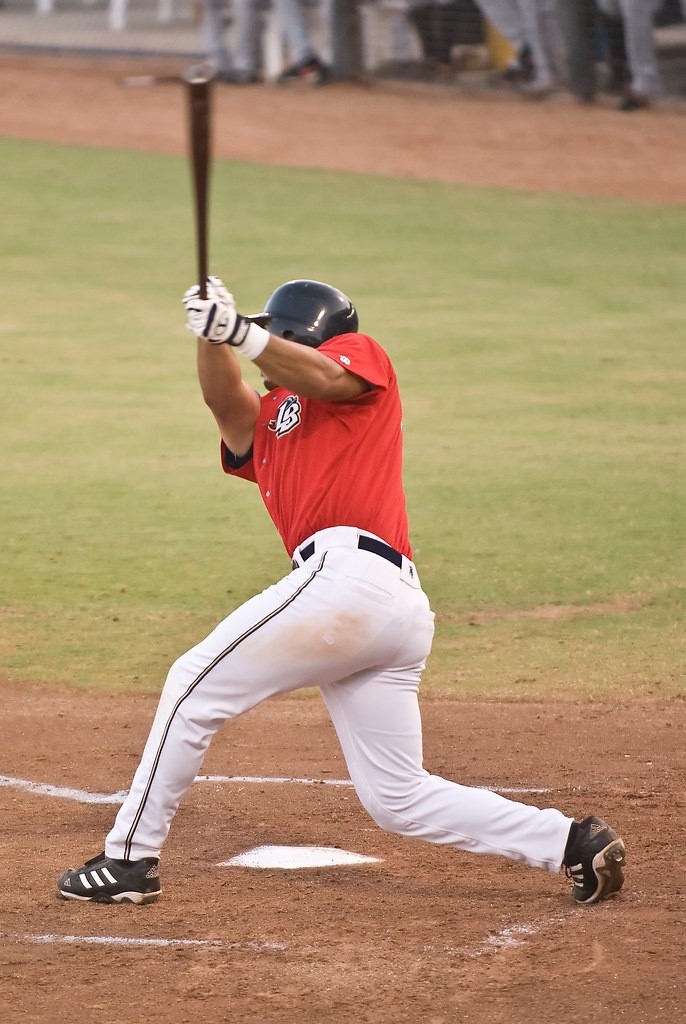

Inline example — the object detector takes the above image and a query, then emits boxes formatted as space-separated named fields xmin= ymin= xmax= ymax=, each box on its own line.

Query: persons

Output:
xmin=58 ymin=278 xmax=626 ymax=907
xmin=189 ymin=0 xmax=667 ymax=112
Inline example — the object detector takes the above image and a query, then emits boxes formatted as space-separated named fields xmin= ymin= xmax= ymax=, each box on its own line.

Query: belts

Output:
xmin=292 ymin=534 xmax=414 ymax=576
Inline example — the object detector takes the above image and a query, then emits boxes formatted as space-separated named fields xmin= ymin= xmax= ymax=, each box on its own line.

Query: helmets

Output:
xmin=244 ymin=280 xmax=359 ymax=348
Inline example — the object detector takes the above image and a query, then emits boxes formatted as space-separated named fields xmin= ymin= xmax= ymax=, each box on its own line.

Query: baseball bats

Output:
xmin=186 ymin=69 xmax=214 ymax=306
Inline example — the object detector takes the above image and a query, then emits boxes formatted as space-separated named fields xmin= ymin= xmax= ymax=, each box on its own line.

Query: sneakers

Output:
xmin=563 ymin=815 xmax=626 ymax=904
xmin=57 ymin=850 xmax=163 ymax=906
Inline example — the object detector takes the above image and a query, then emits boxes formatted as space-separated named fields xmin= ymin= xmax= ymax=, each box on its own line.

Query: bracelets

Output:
xmin=234 ymin=322 xmax=270 ymax=361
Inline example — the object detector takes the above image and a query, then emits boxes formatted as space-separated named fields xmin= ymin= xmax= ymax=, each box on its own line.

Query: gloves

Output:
xmin=182 ymin=276 xmax=234 ymax=318
xmin=185 ymin=275 xmax=271 ymax=360
